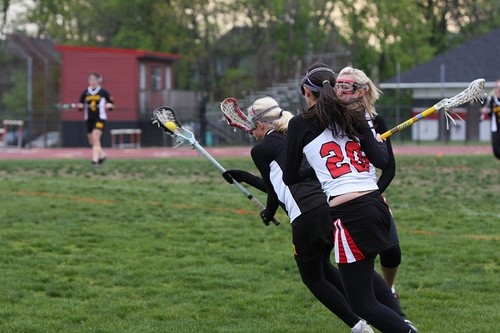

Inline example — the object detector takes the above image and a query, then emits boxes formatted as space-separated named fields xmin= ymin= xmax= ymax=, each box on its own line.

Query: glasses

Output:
xmin=248 ymin=115 xmax=260 ymax=129
xmin=335 ymin=80 xmax=368 ymax=94
xmin=298 ymin=76 xmax=309 ymax=95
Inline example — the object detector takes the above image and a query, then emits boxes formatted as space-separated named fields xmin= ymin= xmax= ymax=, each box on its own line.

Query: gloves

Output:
xmin=222 ymin=170 xmax=248 ymax=184
xmin=260 ymin=209 xmax=274 ymax=226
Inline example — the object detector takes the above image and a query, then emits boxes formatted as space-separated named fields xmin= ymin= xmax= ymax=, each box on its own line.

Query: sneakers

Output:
xmin=391 ymin=286 xmax=398 ymax=301
xmin=404 ymin=319 xmax=417 ymax=332
xmin=350 ymin=323 xmax=375 ymax=333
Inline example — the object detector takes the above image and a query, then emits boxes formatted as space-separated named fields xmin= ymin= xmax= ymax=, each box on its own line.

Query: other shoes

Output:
xmin=92 ymin=159 xmax=98 ymax=164
xmin=99 ymin=156 xmax=106 ymax=163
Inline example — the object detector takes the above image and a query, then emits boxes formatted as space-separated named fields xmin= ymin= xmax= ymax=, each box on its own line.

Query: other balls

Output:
xmin=165 ymin=122 xmax=176 ymax=131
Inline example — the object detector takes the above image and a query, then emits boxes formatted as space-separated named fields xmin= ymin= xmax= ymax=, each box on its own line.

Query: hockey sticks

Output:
xmin=221 ymin=96 xmax=256 ymax=135
xmin=55 ymin=103 xmax=105 ymax=109
xmin=152 ymin=106 xmax=281 ymax=226
xmin=382 ymin=78 xmax=486 ymax=142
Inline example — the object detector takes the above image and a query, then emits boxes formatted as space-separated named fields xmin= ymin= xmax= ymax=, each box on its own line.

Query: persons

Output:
xmin=282 ymin=64 xmax=419 ymax=333
xmin=480 ymin=80 xmax=500 ymax=159
xmin=334 ymin=66 xmax=401 ymax=299
xmin=76 ymin=72 xmax=116 ymax=165
xmin=222 ymin=96 xmax=374 ymax=333
